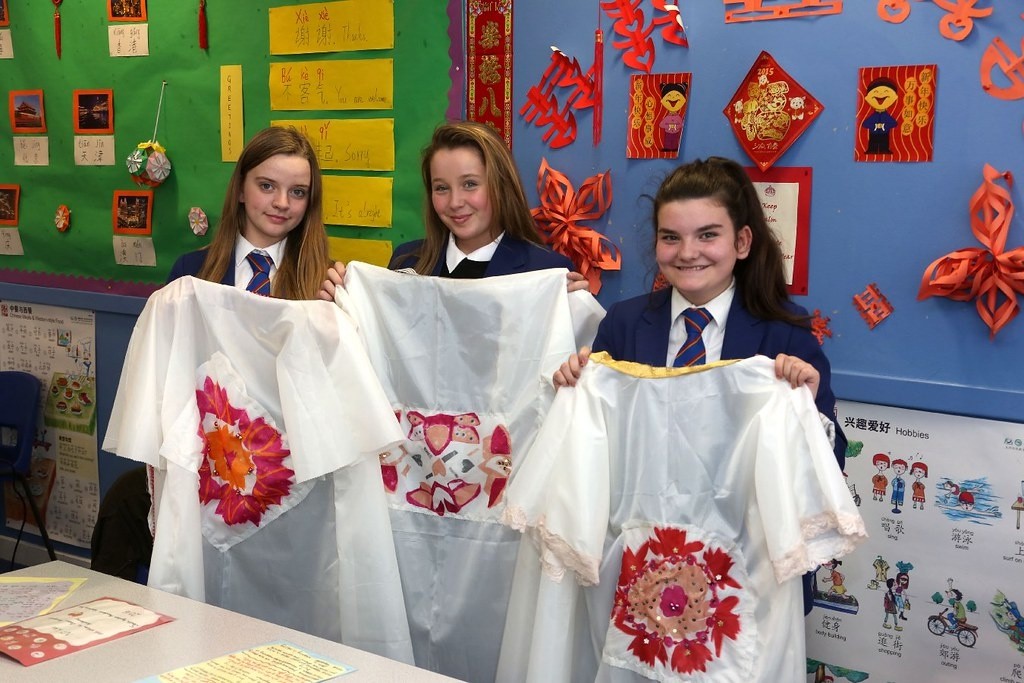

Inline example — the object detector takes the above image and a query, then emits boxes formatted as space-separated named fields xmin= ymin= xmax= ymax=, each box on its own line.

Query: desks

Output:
xmin=0 ymin=559 xmax=469 ymax=683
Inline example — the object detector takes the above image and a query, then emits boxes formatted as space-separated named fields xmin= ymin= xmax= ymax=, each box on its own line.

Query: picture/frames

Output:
xmin=0 ymin=183 xmax=21 ymax=226
xmin=8 ymin=88 xmax=47 ymax=134
xmin=73 ymin=88 xmax=114 ymax=134
xmin=0 ymin=0 xmax=10 ymax=26
xmin=107 ymin=0 xmax=148 ymax=21
xmin=113 ymin=189 xmax=153 ymax=235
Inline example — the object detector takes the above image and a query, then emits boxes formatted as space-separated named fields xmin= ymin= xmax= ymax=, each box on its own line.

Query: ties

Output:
xmin=672 ymin=307 xmax=712 ymax=368
xmin=244 ymin=253 xmax=273 ymax=297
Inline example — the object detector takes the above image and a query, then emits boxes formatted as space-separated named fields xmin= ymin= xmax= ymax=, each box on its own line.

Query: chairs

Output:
xmin=0 ymin=370 xmax=59 ymax=562
xmin=87 ymin=466 xmax=153 ymax=585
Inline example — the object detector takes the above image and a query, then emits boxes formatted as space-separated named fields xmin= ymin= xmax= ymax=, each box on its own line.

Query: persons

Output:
xmin=553 ymin=156 xmax=849 ymax=472
xmin=166 ymin=126 xmax=336 ymax=300
xmin=320 ymin=124 xmax=589 ymax=302
